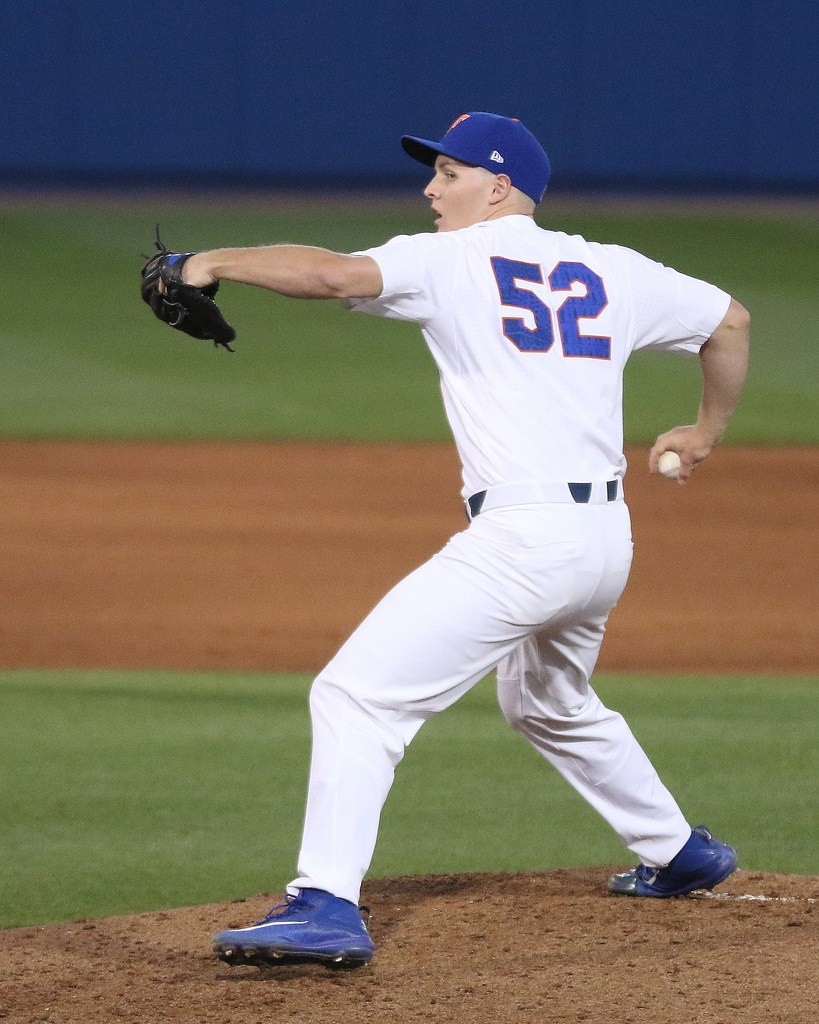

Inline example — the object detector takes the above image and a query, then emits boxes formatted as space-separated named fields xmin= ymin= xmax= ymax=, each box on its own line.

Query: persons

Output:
xmin=127 ymin=111 xmax=751 ymax=974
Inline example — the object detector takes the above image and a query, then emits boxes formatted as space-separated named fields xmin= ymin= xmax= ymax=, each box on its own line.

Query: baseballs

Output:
xmin=658 ymin=451 xmax=692 ymax=478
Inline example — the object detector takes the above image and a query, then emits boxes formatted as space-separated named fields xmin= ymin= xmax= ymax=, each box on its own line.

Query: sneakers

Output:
xmin=609 ymin=828 xmax=738 ymax=896
xmin=209 ymin=890 xmax=374 ymax=971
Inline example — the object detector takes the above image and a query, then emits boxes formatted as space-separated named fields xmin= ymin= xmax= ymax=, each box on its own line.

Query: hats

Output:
xmin=400 ymin=111 xmax=549 ymax=203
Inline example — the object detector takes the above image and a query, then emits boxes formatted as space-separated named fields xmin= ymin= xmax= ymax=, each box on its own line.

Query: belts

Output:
xmin=467 ymin=479 xmax=624 ymax=520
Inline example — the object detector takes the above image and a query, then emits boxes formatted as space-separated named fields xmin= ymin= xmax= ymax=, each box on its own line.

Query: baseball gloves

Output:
xmin=140 ymin=223 xmax=237 ymax=354
